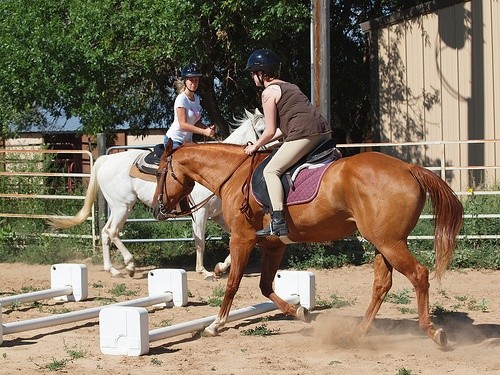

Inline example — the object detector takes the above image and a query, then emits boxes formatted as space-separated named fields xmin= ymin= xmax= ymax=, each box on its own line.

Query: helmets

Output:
xmin=243 ymin=49 xmax=281 ymax=70
xmin=180 ymin=64 xmax=204 ymax=77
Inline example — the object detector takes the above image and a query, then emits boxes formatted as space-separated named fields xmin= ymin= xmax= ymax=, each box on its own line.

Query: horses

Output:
xmin=150 ymin=138 xmax=465 ymax=348
xmin=44 ymin=108 xmax=280 ymax=282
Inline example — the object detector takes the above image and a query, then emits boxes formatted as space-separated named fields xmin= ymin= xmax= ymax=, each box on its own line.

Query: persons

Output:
xmin=151 ymin=63 xmax=215 ymax=220
xmin=242 ymin=49 xmax=332 ymax=238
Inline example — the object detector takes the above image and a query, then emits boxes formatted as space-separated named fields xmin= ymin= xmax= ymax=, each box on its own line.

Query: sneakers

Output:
xmin=256 ymin=219 xmax=289 ymax=235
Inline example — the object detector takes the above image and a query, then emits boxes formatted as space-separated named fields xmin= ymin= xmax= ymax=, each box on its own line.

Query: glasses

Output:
xmin=250 ymin=70 xmax=255 ymax=78
